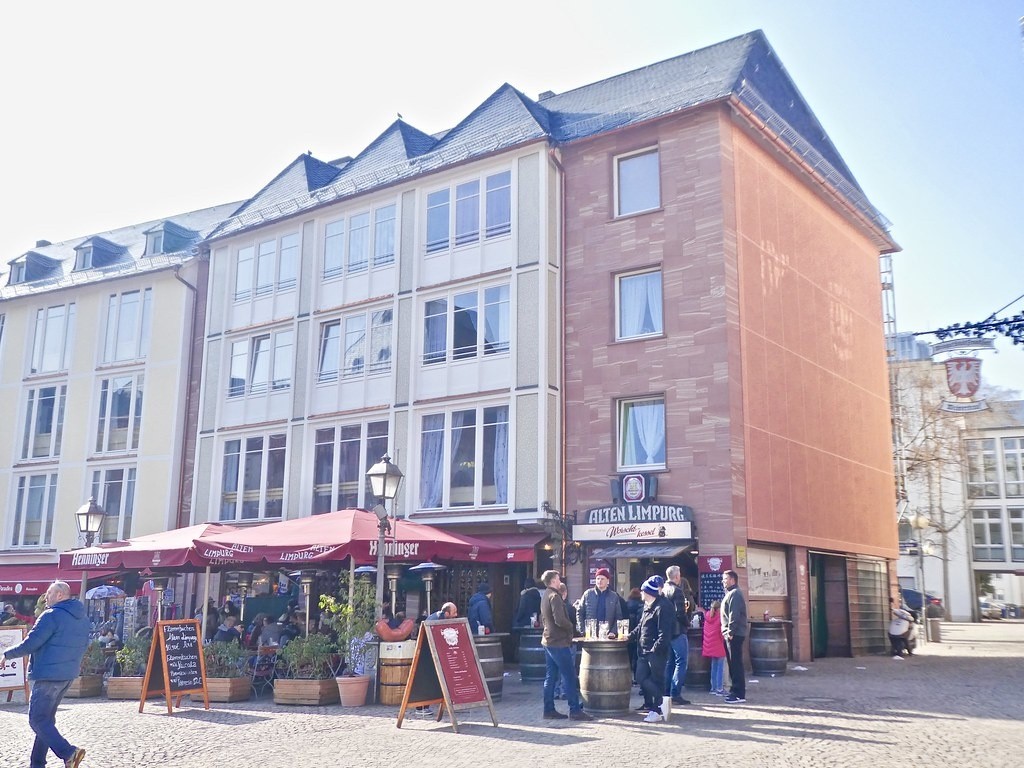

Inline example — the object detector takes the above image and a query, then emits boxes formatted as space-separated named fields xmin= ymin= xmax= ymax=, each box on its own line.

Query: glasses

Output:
xmin=237 ymin=625 xmax=244 ymax=629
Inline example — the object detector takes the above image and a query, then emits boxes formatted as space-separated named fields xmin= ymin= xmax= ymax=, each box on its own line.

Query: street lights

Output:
xmin=74 ymin=496 xmax=106 ymax=607
xmin=365 ymin=452 xmax=405 ymax=699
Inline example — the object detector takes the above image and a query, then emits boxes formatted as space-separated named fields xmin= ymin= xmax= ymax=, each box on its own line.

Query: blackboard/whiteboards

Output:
xmin=143 ymin=619 xmax=206 ymax=696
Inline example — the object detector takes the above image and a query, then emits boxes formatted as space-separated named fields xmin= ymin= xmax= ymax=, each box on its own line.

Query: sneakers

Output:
xmin=716 ymin=689 xmax=731 ymax=696
xmin=643 ymin=710 xmax=653 ymax=722
xmin=709 ymin=688 xmax=717 ymax=694
xmin=725 ymin=694 xmax=746 ymax=703
xmin=648 ymin=712 xmax=662 ymax=722
xmin=65 ymin=747 xmax=85 ymax=768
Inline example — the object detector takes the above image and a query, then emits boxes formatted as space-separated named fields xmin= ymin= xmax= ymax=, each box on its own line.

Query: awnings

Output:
xmin=0 ymin=562 xmax=130 ymax=595
xmin=589 ymin=541 xmax=698 ymax=560
xmin=461 ymin=532 xmax=551 ymax=563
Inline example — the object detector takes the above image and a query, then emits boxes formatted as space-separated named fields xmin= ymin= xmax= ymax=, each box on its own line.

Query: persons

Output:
xmin=619 ymin=574 xmax=675 ymax=724
xmin=378 ymin=596 xmax=427 ymax=639
xmin=576 ymin=568 xmax=622 ymax=709
xmin=538 ymin=570 xmax=593 ymax=721
xmin=609 ymin=585 xmax=630 ymax=620
xmin=623 ymin=587 xmax=644 ymax=687
xmin=0 ymin=604 xmax=14 ymax=626
xmin=660 ymin=566 xmax=692 ymax=705
xmin=97 ymin=631 xmax=124 ymax=676
xmin=719 ymin=570 xmax=750 ymax=704
xmin=413 ymin=601 xmax=459 ymax=717
xmin=0 ymin=582 xmax=92 ymax=768
xmin=194 ymin=591 xmax=349 ymax=672
xmin=466 ymin=583 xmax=496 ymax=634
xmin=513 ymin=577 xmax=541 ymax=627
xmin=553 ymin=582 xmax=578 ymax=700
xmin=700 ymin=600 xmax=732 ymax=698
xmin=678 ymin=577 xmax=695 ymax=628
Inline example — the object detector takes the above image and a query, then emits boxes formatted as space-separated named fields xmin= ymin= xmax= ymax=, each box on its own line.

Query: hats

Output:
xmin=641 ymin=575 xmax=664 ymax=597
xmin=595 ymin=570 xmax=610 ymax=579
xmin=477 ymin=583 xmax=493 ymax=594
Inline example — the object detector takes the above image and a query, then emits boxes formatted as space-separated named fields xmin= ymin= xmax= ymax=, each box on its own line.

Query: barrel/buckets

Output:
xmin=577 ymin=641 xmax=632 ymax=718
xmin=685 ymin=627 xmax=711 ymax=692
xmin=474 ymin=636 xmax=503 ymax=702
xmin=380 ymin=655 xmax=414 ymax=707
xmin=518 ymin=630 xmax=546 ymax=682
xmin=748 ymin=622 xmax=789 ymax=677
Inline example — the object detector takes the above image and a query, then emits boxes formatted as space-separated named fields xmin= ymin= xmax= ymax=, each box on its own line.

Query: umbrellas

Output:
xmin=57 ymin=521 xmax=246 ymax=643
xmin=191 ymin=507 xmax=509 ymax=665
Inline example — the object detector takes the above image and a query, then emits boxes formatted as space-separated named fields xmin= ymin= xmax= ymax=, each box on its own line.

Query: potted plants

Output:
xmin=64 ymin=640 xmax=105 ymax=698
xmin=273 ymin=633 xmax=342 ymax=706
xmin=190 ymin=637 xmax=252 ymax=703
xmin=107 ymin=636 xmax=162 ymax=698
xmin=318 ymin=569 xmax=390 ymax=707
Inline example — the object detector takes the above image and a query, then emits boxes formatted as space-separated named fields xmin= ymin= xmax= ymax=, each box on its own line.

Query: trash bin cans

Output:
xmin=926 ymin=617 xmax=941 ymax=642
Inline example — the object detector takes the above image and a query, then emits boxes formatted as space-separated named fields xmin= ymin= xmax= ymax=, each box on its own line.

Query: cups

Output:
xmin=477 ymin=625 xmax=486 ymax=636
xmin=530 ymin=612 xmax=543 ymax=628
xmin=583 ymin=618 xmax=630 ymax=641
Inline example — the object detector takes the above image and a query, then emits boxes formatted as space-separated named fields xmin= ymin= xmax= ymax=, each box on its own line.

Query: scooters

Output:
xmin=886 ymin=607 xmax=917 ymax=656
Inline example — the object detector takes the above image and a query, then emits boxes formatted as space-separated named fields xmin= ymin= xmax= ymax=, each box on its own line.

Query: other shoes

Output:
xmin=415 ymin=710 xmax=427 ymax=715
xmin=671 ymin=696 xmax=691 ymax=705
xmin=543 ymin=708 xmax=568 ymax=719
xmin=635 ymin=704 xmax=652 ymax=712
xmin=424 ymin=709 xmax=433 ymax=715
xmin=570 ymin=711 xmax=594 ymax=720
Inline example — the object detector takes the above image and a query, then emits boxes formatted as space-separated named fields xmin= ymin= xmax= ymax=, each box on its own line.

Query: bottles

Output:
xmin=763 ymin=614 xmax=769 ymax=621
